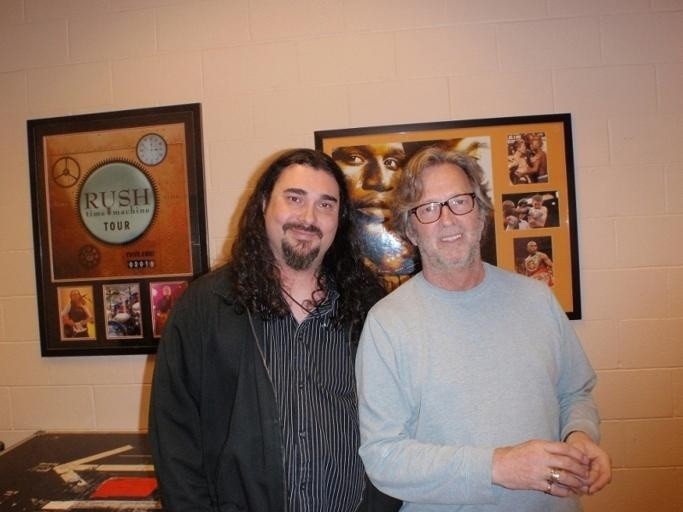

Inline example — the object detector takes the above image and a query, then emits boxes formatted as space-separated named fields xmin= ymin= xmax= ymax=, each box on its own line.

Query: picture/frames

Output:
xmin=313 ymin=112 xmax=581 ymax=319
xmin=26 ymin=102 xmax=208 ymax=357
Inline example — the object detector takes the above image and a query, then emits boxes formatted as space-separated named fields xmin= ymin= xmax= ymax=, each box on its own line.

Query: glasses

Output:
xmin=407 ymin=193 xmax=477 ymax=224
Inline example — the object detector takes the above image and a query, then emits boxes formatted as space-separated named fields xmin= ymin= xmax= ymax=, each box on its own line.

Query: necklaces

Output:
xmin=278 ymin=287 xmax=328 ymax=331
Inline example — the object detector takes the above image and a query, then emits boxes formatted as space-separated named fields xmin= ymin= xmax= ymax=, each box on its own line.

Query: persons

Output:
xmin=154 ymin=293 xmax=175 ymax=335
xmin=349 ymin=147 xmax=613 ymax=511
xmin=131 ymin=292 xmax=142 ymax=335
xmin=148 ymin=149 xmax=401 ymax=512
xmin=59 ymin=289 xmax=90 ymax=341
xmin=332 ymin=140 xmax=415 ymax=288
xmin=500 ymin=136 xmax=561 ymax=284
xmin=105 ymin=292 xmax=132 ymax=337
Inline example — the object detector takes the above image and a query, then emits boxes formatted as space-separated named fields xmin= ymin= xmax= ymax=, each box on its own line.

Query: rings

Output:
xmin=549 ymin=469 xmax=562 ymax=485
xmin=545 ymin=482 xmax=556 ymax=494
